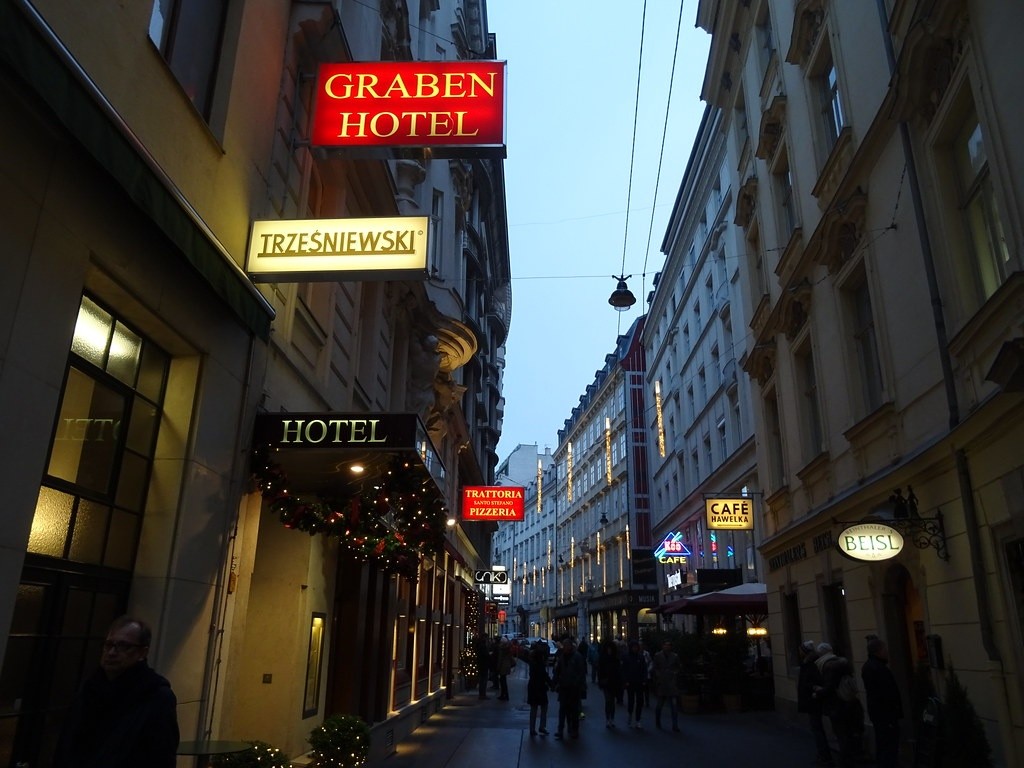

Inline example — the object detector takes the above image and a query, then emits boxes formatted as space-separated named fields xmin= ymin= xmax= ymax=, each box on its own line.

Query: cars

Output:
xmin=519 ymin=637 xmax=544 ymax=662
xmin=538 ymin=639 xmax=559 ymax=666
xmin=502 ymin=633 xmax=524 ymax=644
xmin=510 ymin=638 xmax=526 ymax=658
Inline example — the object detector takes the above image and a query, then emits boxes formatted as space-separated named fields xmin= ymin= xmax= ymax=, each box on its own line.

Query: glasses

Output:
xmin=101 ymin=639 xmax=141 ymax=653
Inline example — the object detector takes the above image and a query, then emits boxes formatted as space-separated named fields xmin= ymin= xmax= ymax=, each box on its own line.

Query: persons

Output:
xmin=52 ymin=617 xmax=180 ymax=768
xmin=861 ymin=634 xmax=904 ymax=768
xmin=797 ymin=640 xmax=865 ymax=768
xmin=476 ymin=633 xmax=683 ymax=741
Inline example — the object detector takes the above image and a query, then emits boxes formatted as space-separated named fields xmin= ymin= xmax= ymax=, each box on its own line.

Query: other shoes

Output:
xmin=539 ymin=729 xmax=550 ymax=735
xmin=530 ymin=732 xmax=537 ymax=735
xmin=554 ymin=733 xmax=563 ymax=738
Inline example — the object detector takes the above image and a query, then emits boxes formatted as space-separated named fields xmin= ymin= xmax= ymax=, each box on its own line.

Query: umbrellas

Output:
xmin=646 ymin=578 xmax=767 ymax=661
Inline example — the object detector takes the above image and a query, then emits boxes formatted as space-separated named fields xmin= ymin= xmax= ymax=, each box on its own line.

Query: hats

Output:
xmin=817 ymin=642 xmax=834 ymax=656
xmin=800 ymin=640 xmax=816 ymax=654
